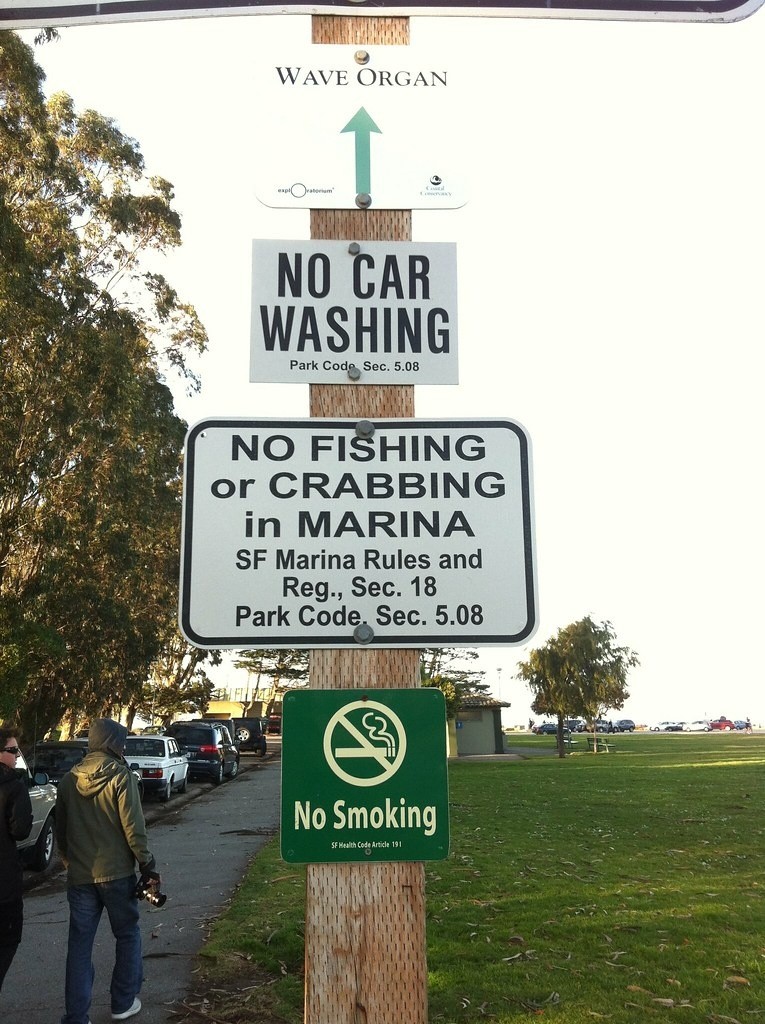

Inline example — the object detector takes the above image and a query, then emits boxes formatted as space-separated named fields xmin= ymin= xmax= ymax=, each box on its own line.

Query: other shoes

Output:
xmin=111 ymin=997 xmax=141 ymax=1020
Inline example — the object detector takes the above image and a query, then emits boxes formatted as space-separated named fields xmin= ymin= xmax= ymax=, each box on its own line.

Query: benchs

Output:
xmin=555 ymin=736 xmax=578 ymax=748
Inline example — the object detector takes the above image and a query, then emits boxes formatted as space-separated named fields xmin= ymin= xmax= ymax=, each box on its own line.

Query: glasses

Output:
xmin=3 ymin=746 xmax=18 ymax=754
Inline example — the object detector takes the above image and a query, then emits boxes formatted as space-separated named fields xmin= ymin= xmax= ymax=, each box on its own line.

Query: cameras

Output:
xmin=134 ymin=875 xmax=167 ymax=908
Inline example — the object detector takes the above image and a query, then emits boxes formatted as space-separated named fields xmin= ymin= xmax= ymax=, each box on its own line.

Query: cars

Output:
xmin=531 ymin=717 xmax=752 ymax=736
xmin=0 ymin=715 xmax=281 ymax=872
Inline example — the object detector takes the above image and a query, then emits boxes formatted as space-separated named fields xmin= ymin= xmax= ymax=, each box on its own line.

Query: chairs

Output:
xmin=587 ymin=737 xmax=616 ymax=752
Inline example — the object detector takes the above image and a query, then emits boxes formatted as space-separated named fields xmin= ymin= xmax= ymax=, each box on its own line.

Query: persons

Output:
xmin=0 ymin=727 xmax=34 ymax=993
xmin=54 ymin=719 xmax=161 ymax=1024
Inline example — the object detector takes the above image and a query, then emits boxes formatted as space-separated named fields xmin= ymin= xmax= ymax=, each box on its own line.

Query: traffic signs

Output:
xmin=254 ymin=42 xmax=467 ymax=213
